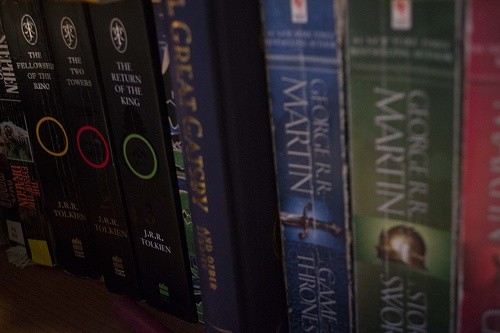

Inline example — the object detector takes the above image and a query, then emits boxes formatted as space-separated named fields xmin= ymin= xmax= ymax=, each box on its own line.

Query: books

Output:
xmin=457 ymin=0 xmax=499 ymax=333
xmin=1 ymin=0 xmax=207 ymax=333
xmin=160 ymin=0 xmax=294 ymax=333
xmin=258 ymin=0 xmax=347 ymax=332
xmin=343 ymin=1 xmax=464 ymax=333
